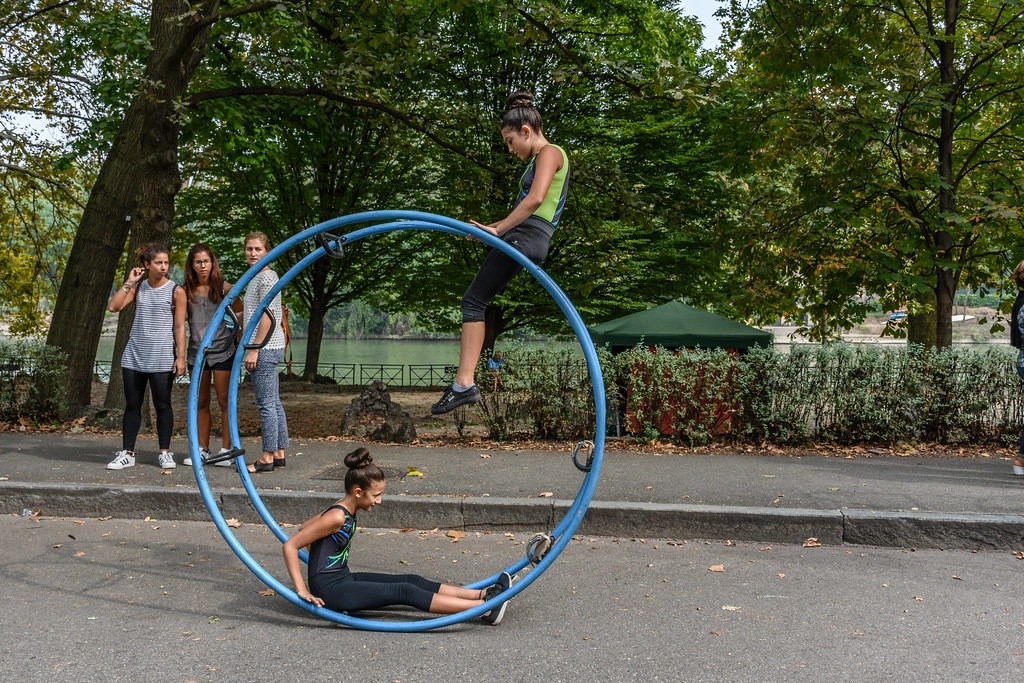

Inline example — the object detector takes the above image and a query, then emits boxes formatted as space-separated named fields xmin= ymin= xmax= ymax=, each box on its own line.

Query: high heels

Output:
xmin=1012 ymin=459 xmax=1023 ymax=475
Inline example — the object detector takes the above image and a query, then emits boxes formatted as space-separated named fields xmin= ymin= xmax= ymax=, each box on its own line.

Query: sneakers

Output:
xmin=431 ymin=384 xmax=482 ymax=414
xmin=158 ymin=451 xmax=176 ymax=468
xmin=214 ymin=448 xmax=235 ymax=467
xmin=481 ymin=572 xmax=519 ymax=625
xmin=183 ymin=446 xmax=211 ymax=466
xmin=108 ymin=450 xmax=136 ymax=469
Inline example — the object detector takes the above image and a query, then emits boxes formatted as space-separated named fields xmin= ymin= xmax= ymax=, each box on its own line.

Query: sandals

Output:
xmin=274 ymin=458 xmax=286 ymax=467
xmin=237 ymin=461 xmax=274 ymax=472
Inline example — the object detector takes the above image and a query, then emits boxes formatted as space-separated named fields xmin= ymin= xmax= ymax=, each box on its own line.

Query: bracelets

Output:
xmin=119 ymin=282 xmax=134 ymax=295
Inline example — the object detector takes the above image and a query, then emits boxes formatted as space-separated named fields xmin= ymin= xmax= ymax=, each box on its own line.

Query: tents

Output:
xmin=585 ymin=301 xmax=775 ymax=439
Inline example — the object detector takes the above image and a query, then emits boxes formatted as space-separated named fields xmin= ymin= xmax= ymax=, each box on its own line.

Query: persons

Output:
xmin=1010 ymin=261 xmax=1023 ymax=477
xmin=428 ymin=91 xmax=571 ymax=415
xmin=178 ymin=243 xmax=245 ymax=467
xmin=237 ymin=229 xmax=287 ymax=474
xmin=105 ymin=242 xmax=187 ymax=469
xmin=282 ymin=448 xmax=511 ymax=625
